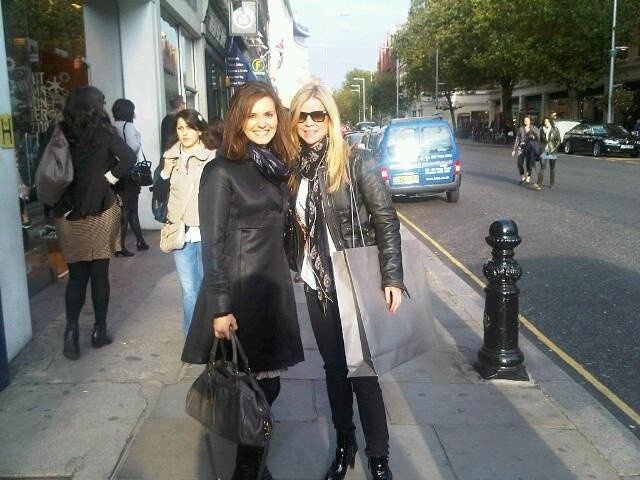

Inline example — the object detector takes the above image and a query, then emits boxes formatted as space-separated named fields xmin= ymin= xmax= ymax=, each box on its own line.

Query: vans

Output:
xmin=371 ymin=115 xmax=461 ymax=202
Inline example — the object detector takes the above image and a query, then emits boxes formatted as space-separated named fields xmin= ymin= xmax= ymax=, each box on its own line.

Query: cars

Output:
xmin=562 ymin=122 xmax=640 ymax=157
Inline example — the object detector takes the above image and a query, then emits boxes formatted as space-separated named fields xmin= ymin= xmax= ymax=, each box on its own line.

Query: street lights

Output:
xmin=380 ymin=47 xmax=399 ymax=117
xmin=349 ymin=77 xmax=365 ymax=122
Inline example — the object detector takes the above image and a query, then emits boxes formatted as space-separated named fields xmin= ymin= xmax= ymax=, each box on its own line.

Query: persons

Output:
xmin=108 ymin=97 xmax=151 ymax=257
xmin=510 ymin=116 xmax=538 ymax=184
xmin=282 ymin=83 xmax=406 ymax=476
xmin=32 ymin=85 xmax=136 ymax=360
xmin=180 ymin=83 xmax=306 ymax=476
xmin=165 ymin=112 xmax=231 ymax=340
xmin=160 ymin=94 xmax=181 ymax=151
xmin=535 ymin=119 xmax=562 ymax=191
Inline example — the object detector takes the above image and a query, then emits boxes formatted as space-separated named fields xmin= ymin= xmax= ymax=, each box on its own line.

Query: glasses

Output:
xmin=298 ymin=110 xmax=328 ymax=123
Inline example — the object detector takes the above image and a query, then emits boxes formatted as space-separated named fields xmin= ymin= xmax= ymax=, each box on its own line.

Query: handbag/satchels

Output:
xmin=331 ymin=161 xmax=440 ymax=379
xmin=34 ymin=123 xmax=73 ymax=204
xmin=184 ymin=327 xmax=273 ymax=448
xmin=159 ymin=164 xmax=202 ymax=254
xmin=122 ymin=123 xmax=155 ymax=187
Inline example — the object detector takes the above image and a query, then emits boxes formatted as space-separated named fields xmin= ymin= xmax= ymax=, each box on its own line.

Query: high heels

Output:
xmin=327 ymin=439 xmax=360 ymax=480
xmin=367 ymin=455 xmax=393 ymax=479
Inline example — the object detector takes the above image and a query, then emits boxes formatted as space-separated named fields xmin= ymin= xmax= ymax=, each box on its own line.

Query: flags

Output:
xmin=251 ymin=56 xmax=275 ymax=84
xmin=231 ymin=44 xmax=256 ymax=88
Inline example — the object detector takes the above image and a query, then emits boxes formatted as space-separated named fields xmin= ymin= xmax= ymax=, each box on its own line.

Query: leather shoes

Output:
xmin=91 ymin=328 xmax=114 ymax=347
xmin=114 ymin=248 xmax=134 ymax=257
xmin=137 ymin=240 xmax=149 ymax=251
xmin=62 ymin=328 xmax=80 ymax=361
